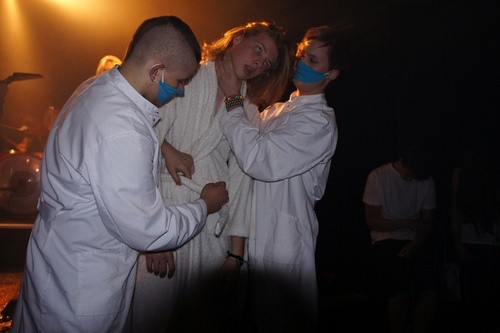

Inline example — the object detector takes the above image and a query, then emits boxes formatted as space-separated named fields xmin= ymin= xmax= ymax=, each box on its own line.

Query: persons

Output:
xmin=3 ymin=113 xmax=44 ymax=164
xmin=95 ymin=55 xmax=122 ymax=75
xmin=363 ymin=142 xmax=437 ymax=333
xmin=213 ymin=22 xmax=342 ymax=333
xmin=10 ymin=17 xmax=231 ymax=333
xmin=123 ymin=16 xmax=293 ymax=333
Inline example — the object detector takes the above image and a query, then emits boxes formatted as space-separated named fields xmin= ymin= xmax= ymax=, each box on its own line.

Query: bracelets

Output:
xmin=227 ymin=249 xmax=245 ymax=266
xmin=224 ymin=93 xmax=246 ymax=112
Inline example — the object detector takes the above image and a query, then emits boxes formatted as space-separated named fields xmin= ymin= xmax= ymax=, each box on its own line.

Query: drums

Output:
xmin=0 ymin=151 xmax=51 ymax=218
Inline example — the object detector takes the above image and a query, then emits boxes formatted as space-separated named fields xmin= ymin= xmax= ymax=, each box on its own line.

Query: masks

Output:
xmin=293 ymin=59 xmax=336 ymax=83
xmin=152 ymin=68 xmax=185 ymax=108
